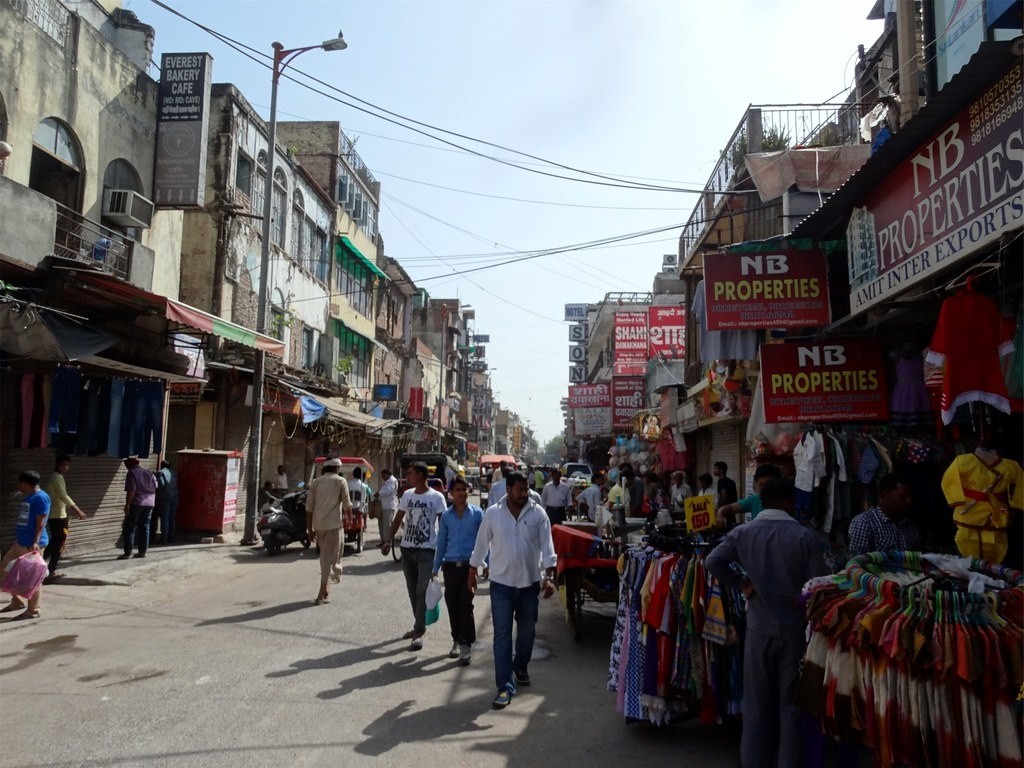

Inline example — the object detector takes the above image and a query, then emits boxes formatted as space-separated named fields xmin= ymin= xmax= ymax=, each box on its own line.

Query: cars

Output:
xmin=466 ymin=467 xmax=487 ymax=487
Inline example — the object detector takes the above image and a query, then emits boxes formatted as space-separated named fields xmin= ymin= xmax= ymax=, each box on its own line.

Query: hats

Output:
xmin=607 ymin=436 xmax=658 ymax=481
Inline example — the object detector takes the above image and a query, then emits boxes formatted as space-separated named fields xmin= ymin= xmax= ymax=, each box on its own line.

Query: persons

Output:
xmin=381 ymin=461 xmax=448 ymax=650
xmin=270 ymin=466 xmax=288 ymax=496
xmin=347 ymin=467 xmax=369 ymax=511
xmin=43 ymin=455 xmax=87 ymax=579
xmin=431 ymin=475 xmax=489 ymax=666
xmin=489 ymin=461 xmax=738 ymax=524
xmin=0 ymin=471 xmax=50 ymax=620
xmin=466 ymin=471 xmax=557 ymax=708
xmin=304 ymin=456 xmax=352 ymax=604
xmin=150 ymin=459 xmax=178 ymax=543
xmin=702 ymin=464 xmax=922 ymax=768
xmin=118 ymin=457 xmax=156 ymax=559
xmin=258 ymin=481 xmax=280 ymax=512
xmin=374 ymin=469 xmax=398 ymax=548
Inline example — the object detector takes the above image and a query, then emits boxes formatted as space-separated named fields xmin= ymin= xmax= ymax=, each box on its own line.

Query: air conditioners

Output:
xmin=662 ymin=254 xmax=678 ymax=273
xmin=102 ymin=188 xmax=155 ymax=229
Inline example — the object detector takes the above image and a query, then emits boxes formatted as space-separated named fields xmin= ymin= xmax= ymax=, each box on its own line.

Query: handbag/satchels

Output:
xmin=0 ymin=550 xmax=49 ymax=599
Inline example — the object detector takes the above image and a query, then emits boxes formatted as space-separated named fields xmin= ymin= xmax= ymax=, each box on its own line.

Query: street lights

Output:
xmin=239 ymin=32 xmax=348 ymax=546
xmin=435 ymin=301 xmax=473 ymax=452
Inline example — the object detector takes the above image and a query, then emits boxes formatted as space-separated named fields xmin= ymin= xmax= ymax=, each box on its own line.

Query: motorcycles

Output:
xmin=397 ymin=451 xmax=466 ymax=506
xmin=479 ymin=455 xmax=518 ymax=510
xmin=305 ymin=454 xmax=374 ymax=551
xmin=258 ymin=466 xmax=311 ymax=553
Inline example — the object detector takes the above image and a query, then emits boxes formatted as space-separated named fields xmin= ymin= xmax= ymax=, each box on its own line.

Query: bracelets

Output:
xmin=469 ymin=570 xmax=477 ymax=575
xmin=546 ymin=576 xmax=554 ymax=579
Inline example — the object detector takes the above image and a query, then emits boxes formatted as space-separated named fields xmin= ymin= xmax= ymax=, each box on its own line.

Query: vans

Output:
xmin=560 ymin=463 xmax=594 ymax=487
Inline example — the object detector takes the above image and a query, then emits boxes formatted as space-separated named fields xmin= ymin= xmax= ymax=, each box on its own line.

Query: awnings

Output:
xmin=67 ymin=268 xmax=283 ymax=359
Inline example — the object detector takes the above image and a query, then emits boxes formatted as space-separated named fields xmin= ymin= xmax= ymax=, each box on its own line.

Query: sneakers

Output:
xmin=450 ymin=641 xmax=460 ymax=657
xmin=513 ymin=664 xmax=529 ymax=684
xmin=12 ymin=608 xmax=41 ymax=620
xmin=0 ymin=605 xmax=25 ymax=613
xmin=491 ymin=682 xmax=516 ymax=705
xmin=460 ymin=645 xmax=471 ymax=664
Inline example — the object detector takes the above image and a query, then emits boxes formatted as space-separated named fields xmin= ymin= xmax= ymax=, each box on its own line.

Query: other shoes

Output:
xmin=322 ymin=590 xmax=330 ymax=602
xmin=118 ymin=555 xmax=129 ymax=559
xmin=404 ymin=631 xmax=416 ymax=638
xmin=134 ymin=553 xmax=145 ymax=558
xmin=316 ymin=599 xmax=323 ymax=605
xmin=412 ymin=633 xmax=422 ymax=649
xmin=48 ymin=572 xmax=65 ymax=580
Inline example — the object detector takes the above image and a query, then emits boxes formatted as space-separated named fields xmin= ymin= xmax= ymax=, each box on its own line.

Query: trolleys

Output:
xmin=551 ymin=517 xmax=653 ymax=643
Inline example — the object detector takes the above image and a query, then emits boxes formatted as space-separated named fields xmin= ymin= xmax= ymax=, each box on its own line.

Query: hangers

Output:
xmin=22 ymin=360 xmax=159 ymax=385
xmin=959 ymin=274 xmax=981 ymax=296
xmin=801 ymin=422 xmax=894 ymax=442
xmin=643 ymin=523 xmax=728 ymax=563
xmin=830 ymin=551 xmax=1024 ymax=627
